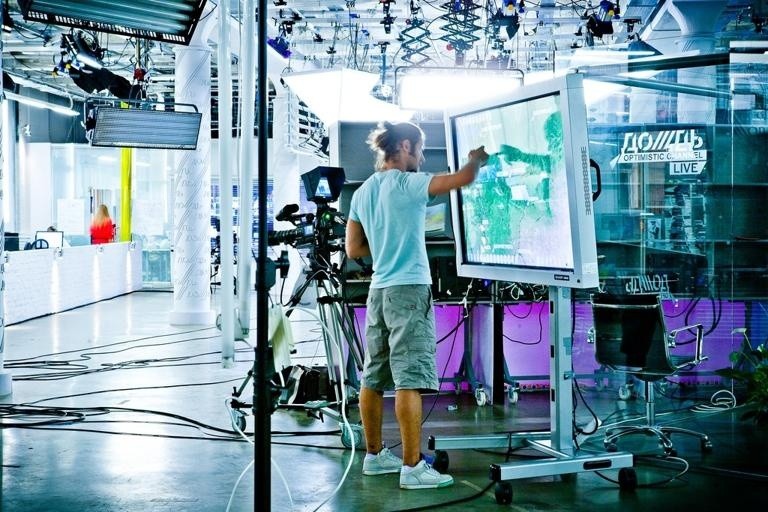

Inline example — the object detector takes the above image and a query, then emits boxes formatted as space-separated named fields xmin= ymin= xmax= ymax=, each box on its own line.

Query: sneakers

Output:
xmin=400 ymin=460 xmax=453 ymax=490
xmin=362 ymin=447 xmax=403 ymax=476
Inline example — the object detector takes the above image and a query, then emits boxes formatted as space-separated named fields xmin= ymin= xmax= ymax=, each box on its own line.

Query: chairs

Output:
xmin=589 ymin=290 xmax=713 ymax=459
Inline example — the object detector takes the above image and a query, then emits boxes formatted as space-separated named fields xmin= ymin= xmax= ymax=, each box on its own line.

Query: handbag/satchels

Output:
xmin=275 ymin=365 xmax=320 ymax=405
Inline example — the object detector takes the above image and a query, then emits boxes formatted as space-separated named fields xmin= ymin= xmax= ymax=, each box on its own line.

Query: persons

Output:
xmin=46 ymin=225 xmax=71 ymax=248
xmin=493 ymin=110 xmax=567 ymax=225
xmin=343 ymin=120 xmax=490 ymax=490
xmin=88 ymin=205 xmax=116 ymax=245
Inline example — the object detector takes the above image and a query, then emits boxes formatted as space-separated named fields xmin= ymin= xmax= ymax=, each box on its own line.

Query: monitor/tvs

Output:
xmin=4 ymin=232 xmax=19 ymax=252
xmin=35 ymin=231 xmax=63 ymax=249
xmin=443 ymin=73 xmax=601 ymax=289
